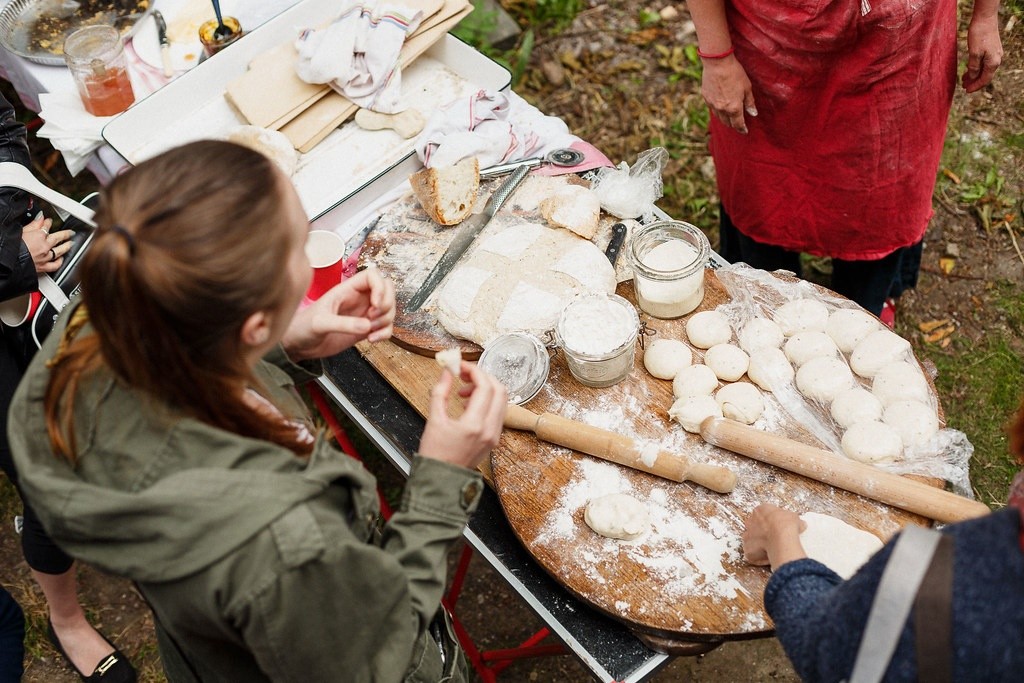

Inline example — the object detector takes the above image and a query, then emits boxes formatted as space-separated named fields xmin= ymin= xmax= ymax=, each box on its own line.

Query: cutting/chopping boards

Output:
xmin=491 ymin=267 xmax=944 ymax=637
xmin=359 ymin=173 xmax=637 ymax=361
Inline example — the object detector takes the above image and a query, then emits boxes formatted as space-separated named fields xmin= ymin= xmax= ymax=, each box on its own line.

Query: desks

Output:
xmin=0 ymin=0 xmax=187 ymax=186
xmin=301 ymin=346 xmax=678 ymax=683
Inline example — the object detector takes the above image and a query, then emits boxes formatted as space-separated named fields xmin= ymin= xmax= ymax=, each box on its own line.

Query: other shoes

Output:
xmin=50 ymin=616 xmax=137 ymax=683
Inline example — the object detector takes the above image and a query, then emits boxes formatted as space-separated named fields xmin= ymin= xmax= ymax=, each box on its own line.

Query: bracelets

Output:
xmin=697 ymin=45 xmax=735 ymax=59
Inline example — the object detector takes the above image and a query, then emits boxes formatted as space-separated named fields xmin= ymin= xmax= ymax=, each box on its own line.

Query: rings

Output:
xmin=50 ymin=247 xmax=58 ymax=261
xmin=40 ymin=228 xmax=53 ymax=237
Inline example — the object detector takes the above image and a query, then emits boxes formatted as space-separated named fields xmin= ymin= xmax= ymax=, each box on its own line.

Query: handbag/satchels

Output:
xmin=0 ymin=162 xmax=103 ymax=348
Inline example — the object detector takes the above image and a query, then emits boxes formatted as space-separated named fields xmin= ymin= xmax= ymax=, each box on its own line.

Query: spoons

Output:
xmin=211 ymin=0 xmax=233 ymax=40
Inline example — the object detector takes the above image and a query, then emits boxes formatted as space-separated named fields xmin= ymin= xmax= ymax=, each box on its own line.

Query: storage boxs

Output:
xmin=98 ymin=0 xmax=514 ymax=234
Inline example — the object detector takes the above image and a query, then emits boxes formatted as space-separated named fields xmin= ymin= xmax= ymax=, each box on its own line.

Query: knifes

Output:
xmin=406 ymin=164 xmax=530 ymax=311
xmin=154 ymin=11 xmax=172 ymax=78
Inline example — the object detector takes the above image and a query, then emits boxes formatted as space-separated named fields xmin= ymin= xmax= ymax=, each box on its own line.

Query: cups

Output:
xmin=304 ymin=230 xmax=345 ymax=301
xmin=62 ymin=25 xmax=135 ymax=117
xmin=0 ymin=290 xmax=43 ymax=327
xmin=198 ymin=15 xmax=244 ymax=56
xmin=628 ymin=221 xmax=711 ymax=318
xmin=556 ymin=293 xmax=639 ymax=387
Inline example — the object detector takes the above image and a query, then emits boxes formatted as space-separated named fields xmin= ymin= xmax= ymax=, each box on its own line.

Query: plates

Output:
xmin=132 ymin=0 xmax=210 ymax=70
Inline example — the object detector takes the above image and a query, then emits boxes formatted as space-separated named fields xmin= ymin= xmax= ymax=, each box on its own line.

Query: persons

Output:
xmin=7 ymin=138 xmax=507 ymax=683
xmin=0 ymin=93 xmax=136 ymax=682
xmin=738 ymin=397 xmax=1024 ymax=683
xmin=685 ymin=0 xmax=1005 ymax=319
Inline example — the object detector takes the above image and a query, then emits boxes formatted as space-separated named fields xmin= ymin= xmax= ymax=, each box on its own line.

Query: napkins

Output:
xmin=34 ymin=85 xmax=123 ymax=177
